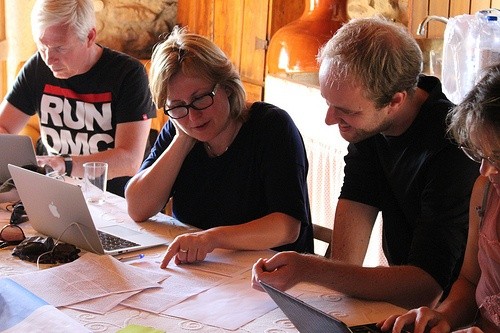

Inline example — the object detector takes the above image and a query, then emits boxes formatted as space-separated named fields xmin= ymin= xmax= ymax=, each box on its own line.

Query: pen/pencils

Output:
xmin=119 ymin=254 xmax=144 ymax=262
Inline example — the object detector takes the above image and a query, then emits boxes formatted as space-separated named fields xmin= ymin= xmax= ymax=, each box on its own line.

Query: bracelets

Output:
xmin=60 ymin=154 xmax=72 ymax=176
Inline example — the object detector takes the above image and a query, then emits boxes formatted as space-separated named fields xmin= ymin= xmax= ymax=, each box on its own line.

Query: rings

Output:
xmin=180 ymin=248 xmax=187 ymax=252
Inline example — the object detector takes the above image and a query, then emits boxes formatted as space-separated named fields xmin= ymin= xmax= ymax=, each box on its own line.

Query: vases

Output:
xmin=266 ymin=0 xmax=352 ymax=75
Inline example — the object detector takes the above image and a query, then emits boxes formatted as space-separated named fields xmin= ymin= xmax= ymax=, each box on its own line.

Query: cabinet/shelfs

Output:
xmin=407 ymin=0 xmax=500 ymax=39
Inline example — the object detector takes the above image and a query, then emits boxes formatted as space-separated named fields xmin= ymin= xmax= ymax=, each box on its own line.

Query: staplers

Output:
xmin=10 ymin=205 xmax=28 ymax=225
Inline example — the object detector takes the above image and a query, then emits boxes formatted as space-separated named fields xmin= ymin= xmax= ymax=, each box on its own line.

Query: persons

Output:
xmin=252 ymin=20 xmax=481 ymax=310
xmin=0 ymin=0 xmax=157 ymax=197
xmin=376 ymin=64 xmax=500 ymax=333
xmin=125 ymin=34 xmax=315 ymax=268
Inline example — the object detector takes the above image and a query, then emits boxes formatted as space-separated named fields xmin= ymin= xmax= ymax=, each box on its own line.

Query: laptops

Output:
xmin=0 ymin=134 xmax=40 ymax=185
xmin=258 ymin=281 xmax=432 ymax=333
xmin=7 ymin=164 xmax=169 ymax=256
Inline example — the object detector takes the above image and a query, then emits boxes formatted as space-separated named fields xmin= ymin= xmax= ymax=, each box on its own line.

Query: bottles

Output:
xmin=475 ymin=16 xmax=500 ymax=85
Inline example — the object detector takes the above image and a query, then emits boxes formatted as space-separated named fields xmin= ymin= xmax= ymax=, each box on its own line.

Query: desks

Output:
xmin=265 ymin=74 xmax=389 ymax=269
xmin=0 ymin=174 xmax=411 ymax=333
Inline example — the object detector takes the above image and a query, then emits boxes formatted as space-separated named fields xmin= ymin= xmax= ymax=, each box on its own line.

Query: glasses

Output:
xmin=163 ymin=81 xmax=219 ymax=119
xmin=0 ymin=224 xmax=26 ymax=243
xmin=461 ymin=143 xmax=500 ymax=169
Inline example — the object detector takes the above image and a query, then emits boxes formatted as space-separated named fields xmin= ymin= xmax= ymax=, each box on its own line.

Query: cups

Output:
xmin=83 ymin=161 xmax=108 ymax=206
